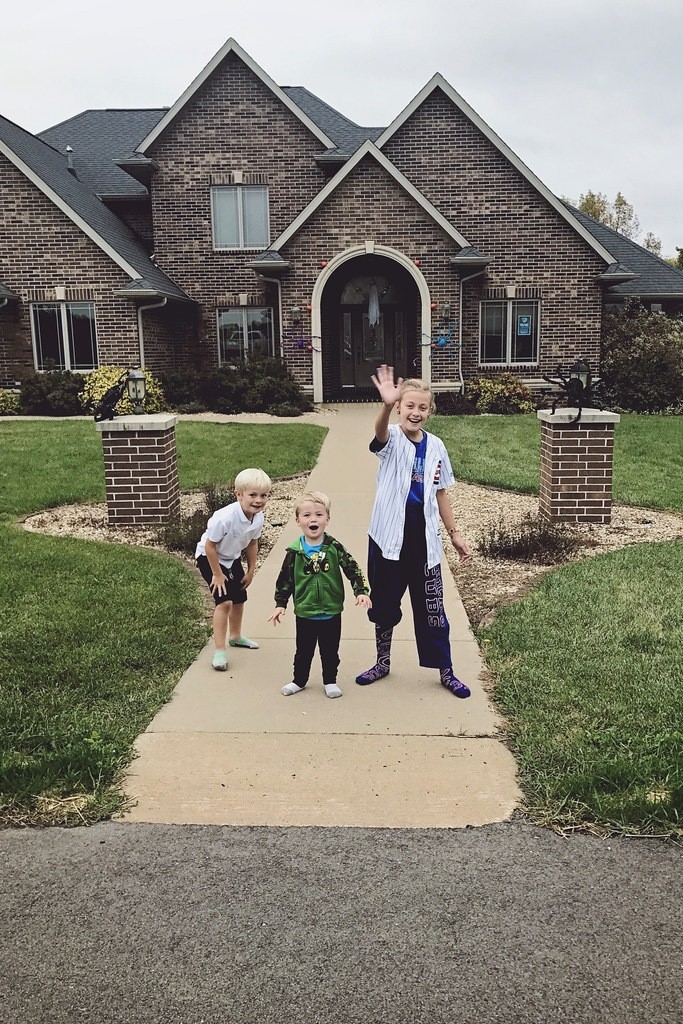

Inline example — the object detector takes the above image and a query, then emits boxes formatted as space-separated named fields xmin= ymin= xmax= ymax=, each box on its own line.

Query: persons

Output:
xmin=194 ymin=468 xmax=271 ymax=670
xmin=356 ymin=364 xmax=471 ymax=699
xmin=268 ymin=492 xmax=372 ymax=699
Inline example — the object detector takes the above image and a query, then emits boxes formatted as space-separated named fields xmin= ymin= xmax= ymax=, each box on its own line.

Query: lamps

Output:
xmin=290 ymin=304 xmax=301 ymax=324
xmin=441 ymin=302 xmax=451 ymax=324
xmin=568 ymin=358 xmax=592 ymax=407
xmin=124 ymin=365 xmax=147 ymax=415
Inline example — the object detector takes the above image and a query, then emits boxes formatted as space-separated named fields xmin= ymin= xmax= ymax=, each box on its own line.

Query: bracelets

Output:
xmin=447 ymin=527 xmax=459 ymax=534
